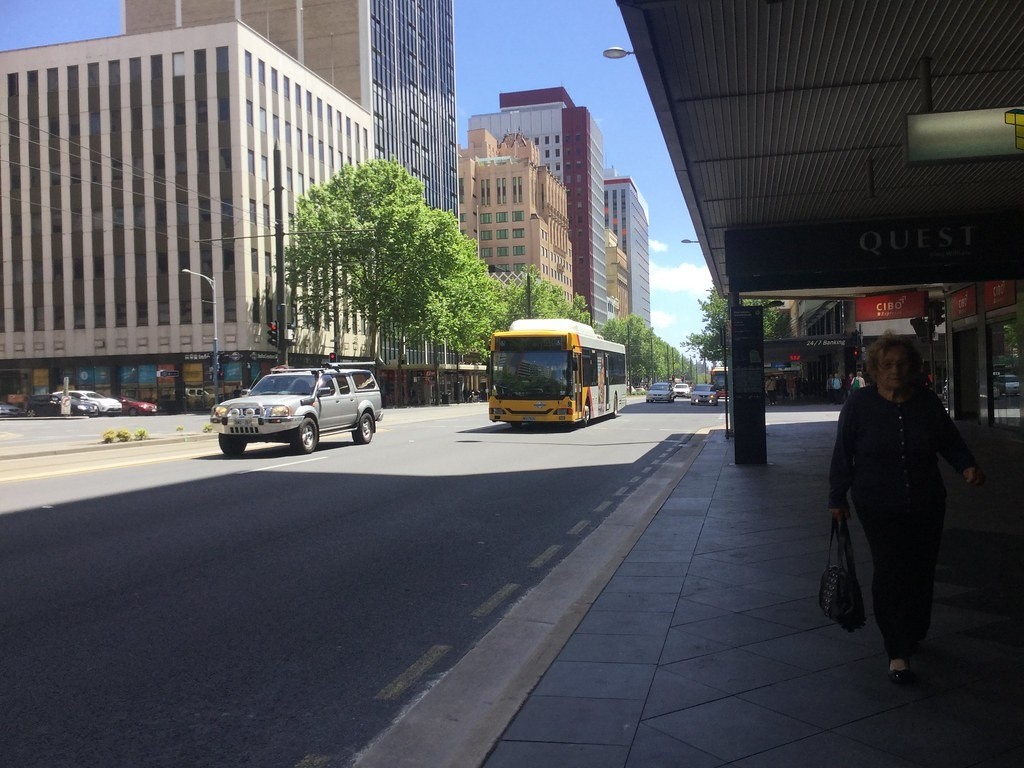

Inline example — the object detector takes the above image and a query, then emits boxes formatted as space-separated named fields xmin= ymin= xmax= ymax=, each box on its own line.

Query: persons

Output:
xmin=846 ymin=373 xmax=854 ymax=396
xmin=826 ymin=374 xmax=834 ymax=399
xmin=851 ymin=371 xmax=865 ymax=388
xmin=827 ymin=337 xmax=982 ymax=678
xmin=831 ymin=373 xmax=842 ymax=401
xmin=766 ymin=376 xmax=777 ymax=405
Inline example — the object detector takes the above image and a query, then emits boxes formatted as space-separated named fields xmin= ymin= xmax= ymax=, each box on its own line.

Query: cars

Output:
xmin=0 ymin=401 xmax=20 ymax=417
xmin=646 ymin=383 xmax=676 ymax=403
xmin=672 ymin=383 xmax=692 ymax=399
xmin=23 ymin=394 xmax=99 ymax=418
xmin=113 ymin=396 xmax=158 ymax=416
xmin=690 ymin=384 xmax=719 ymax=406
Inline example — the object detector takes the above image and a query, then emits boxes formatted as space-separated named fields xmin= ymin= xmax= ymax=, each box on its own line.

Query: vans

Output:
xmin=995 ymin=374 xmax=1019 ymax=396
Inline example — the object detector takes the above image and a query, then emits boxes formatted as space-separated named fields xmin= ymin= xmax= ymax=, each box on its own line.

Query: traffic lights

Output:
xmin=854 ymin=347 xmax=859 ymax=360
xmin=909 ymin=315 xmax=923 ymax=338
xmin=267 ymin=321 xmax=280 ymax=348
xmin=329 ymin=352 xmax=337 ymax=363
xmin=933 ymin=301 xmax=946 ymax=327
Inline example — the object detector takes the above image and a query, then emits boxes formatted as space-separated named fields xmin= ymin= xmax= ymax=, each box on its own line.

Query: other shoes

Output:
xmin=889 ymin=657 xmax=910 ymax=684
xmin=911 ymin=640 xmax=921 ymax=653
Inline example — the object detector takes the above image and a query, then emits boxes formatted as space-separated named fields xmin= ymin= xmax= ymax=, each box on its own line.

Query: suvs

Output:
xmin=173 ymin=387 xmax=208 ymax=398
xmin=52 ymin=390 xmax=123 ymax=417
xmin=210 ymin=367 xmax=385 ymax=455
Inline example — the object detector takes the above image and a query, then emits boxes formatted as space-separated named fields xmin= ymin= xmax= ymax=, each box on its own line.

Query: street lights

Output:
xmin=181 ymin=268 xmax=219 ymax=404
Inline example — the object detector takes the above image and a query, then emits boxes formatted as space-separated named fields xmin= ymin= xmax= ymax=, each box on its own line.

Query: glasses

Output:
xmin=877 ymin=359 xmax=910 ymax=371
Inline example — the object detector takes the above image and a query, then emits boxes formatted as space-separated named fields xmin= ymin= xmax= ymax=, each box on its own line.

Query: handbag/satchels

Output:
xmin=819 ymin=512 xmax=866 ymax=632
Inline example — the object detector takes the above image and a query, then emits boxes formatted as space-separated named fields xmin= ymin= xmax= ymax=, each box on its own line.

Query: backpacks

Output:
xmin=852 ymin=377 xmax=860 ymax=393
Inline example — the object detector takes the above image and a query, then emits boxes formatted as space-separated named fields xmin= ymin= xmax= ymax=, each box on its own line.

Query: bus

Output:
xmin=711 ymin=366 xmax=730 ymax=399
xmin=485 ymin=319 xmax=628 ymax=428
